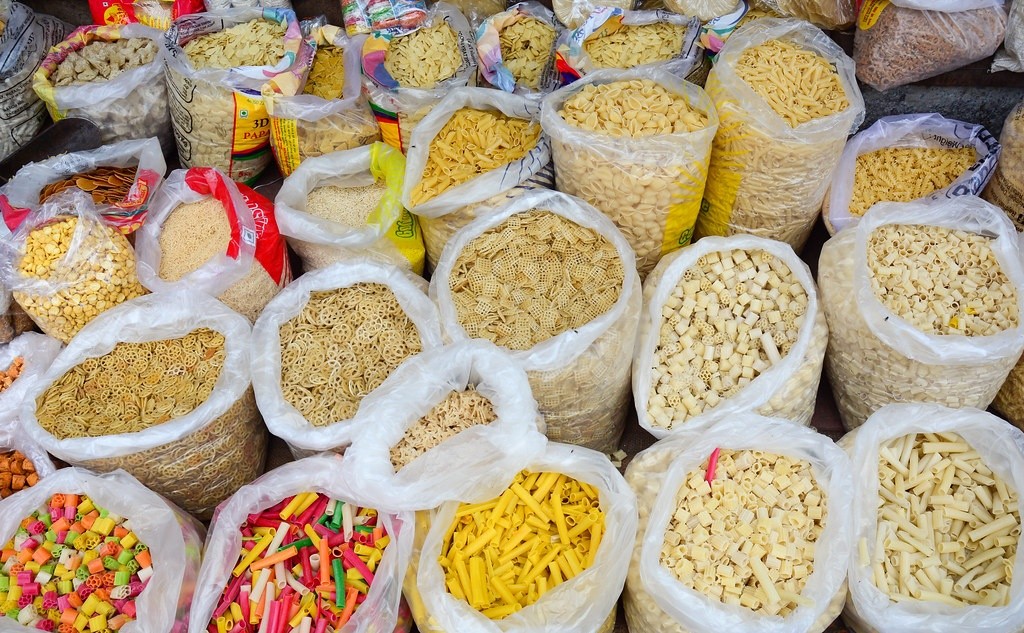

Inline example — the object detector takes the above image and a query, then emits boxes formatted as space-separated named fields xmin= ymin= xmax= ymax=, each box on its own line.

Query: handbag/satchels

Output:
xmin=1 ymin=0 xmax=1022 ymax=632
